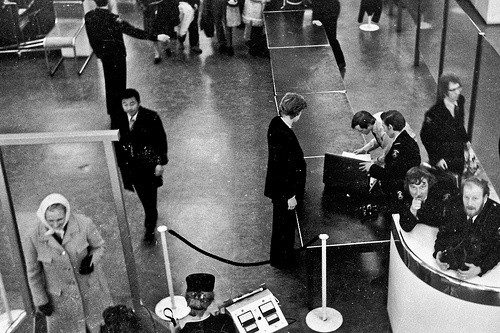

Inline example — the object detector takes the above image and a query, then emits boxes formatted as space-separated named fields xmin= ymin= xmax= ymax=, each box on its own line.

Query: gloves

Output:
xmin=39 ymin=304 xmax=55 ymax=317
xmin=79 ymin=257 xmax=93 ymax=274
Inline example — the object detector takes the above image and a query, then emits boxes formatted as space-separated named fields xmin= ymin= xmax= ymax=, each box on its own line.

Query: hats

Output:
xmin=186 ymin=273 xmax=215 ymax=292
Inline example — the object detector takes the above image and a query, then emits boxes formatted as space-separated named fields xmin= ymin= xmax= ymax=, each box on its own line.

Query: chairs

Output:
xmin=43 ymin=0 xmax=94 ymax=76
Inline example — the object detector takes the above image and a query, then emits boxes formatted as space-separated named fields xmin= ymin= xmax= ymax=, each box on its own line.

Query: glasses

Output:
xmin=448 ymin=86 xmax=462 ymax=93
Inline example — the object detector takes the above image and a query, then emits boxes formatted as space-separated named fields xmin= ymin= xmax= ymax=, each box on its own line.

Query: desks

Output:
xmin=261 ymin=9 xmax=391 ymax=309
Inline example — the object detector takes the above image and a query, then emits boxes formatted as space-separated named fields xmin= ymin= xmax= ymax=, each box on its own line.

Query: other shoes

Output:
xmin=154 ymin=54 xmax=160 ymax=64
xmin=144 ymin=227 xmax=155 ymax=243
xmin=218 ymin=43 xmax=226 ymax=53
xmin=178 ymin=43 xmax=184 ymax=50
xmin=191 ymin=46 xmax=202 ymax=54
xmin=165 ymin=49 xmax=171 ymax=55
xmin=228 ymin=47 xmax=234 ymax=55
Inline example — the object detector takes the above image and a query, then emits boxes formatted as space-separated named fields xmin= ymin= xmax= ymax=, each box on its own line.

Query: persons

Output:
xmin=110 ymin=88 xmax=169 ymax=248
xmin=350 ymin=109 xmax=432 ymax=168
xmin=169 ymin=272 xmax=221 ymax=333
xmin=21 ymin=192 xmax=115 ymax=333
xmin=357 ymin=109 xmax=423 ymax=209
xmin=264 ymin=92 xmax=309 ymax=274
xmin=240 ymin=0 xmax=272 ymax=45
xmin=83 ymin=0 xmax=171 ymax=133
xmin=136 ymin=0 xmax=203 ymax=65
xmin=432 ymin=177 xmax=500 ymax=282
xmin=312 ymin=0 xmax=347 ymax=70
xmin=214 ymin=0 xmax=235 ymax=57
xmin=398 ymin=165 xmax=458 ymax=233
xmin=419 ymin=71 xmax=471 ymax=172
